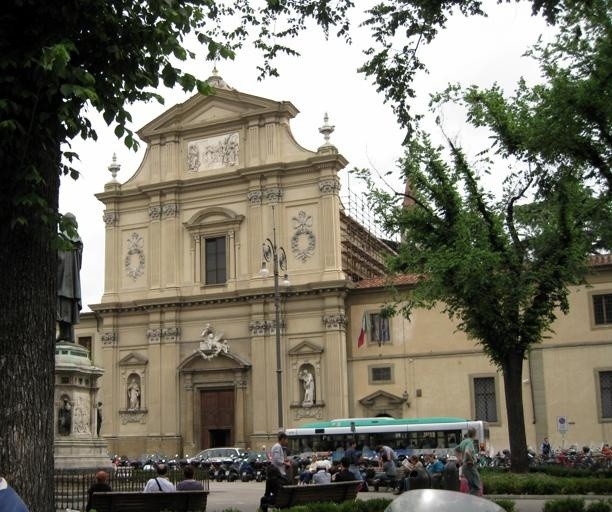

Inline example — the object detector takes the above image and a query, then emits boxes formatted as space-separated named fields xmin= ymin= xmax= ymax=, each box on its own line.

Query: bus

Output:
xmin=280 ymin=416 xmax=492 ymax=463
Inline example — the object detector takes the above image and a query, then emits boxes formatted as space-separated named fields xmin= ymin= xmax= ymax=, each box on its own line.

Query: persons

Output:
xmin=299 ymin=370 xmax=314 ymax=406
xmin=60 ymin=398 xmax=71 ymax=436
xmin=128 ymin=378 xmax=140 ymax=410
xmin=143 ymin=464 xmax=175 ymax=492
xmin=0 ymin=475 xmax=28 ymax=512
xmin=94 ymin=401 xmax=104 ymax=436
xmin=541 ymin=437 xmax=552 ymax=459
xmin=56 ymin=212 xmax=83 ymax=343
xmin=247 ymin=427 xmax=490 ymax=512
xmin=86 ymin=471 xmax=112 ymax=512
xmin=176 ymin=465 xmax=205 ymax=490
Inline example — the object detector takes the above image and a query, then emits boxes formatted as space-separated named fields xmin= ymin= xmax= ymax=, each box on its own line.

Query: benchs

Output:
xmin=265 ymin=479 xmax=363 ymax=512
xmin=330 ymin=465 xmax=407 ymax=490
xmin=85 ymin=490 xmax=209 ymax=512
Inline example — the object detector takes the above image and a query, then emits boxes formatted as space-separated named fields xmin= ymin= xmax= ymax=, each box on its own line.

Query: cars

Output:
xmin=188 ymin=447 xmax=248 ymax=463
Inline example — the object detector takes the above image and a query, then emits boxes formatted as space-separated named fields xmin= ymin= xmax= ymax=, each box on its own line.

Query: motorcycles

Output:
xmin=111 ymin=453 xmax=271 ymax=483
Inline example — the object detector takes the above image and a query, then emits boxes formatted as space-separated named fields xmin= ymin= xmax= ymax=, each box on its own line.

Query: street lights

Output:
xmin=259 ymin=227 xmax=290 ymax=434
